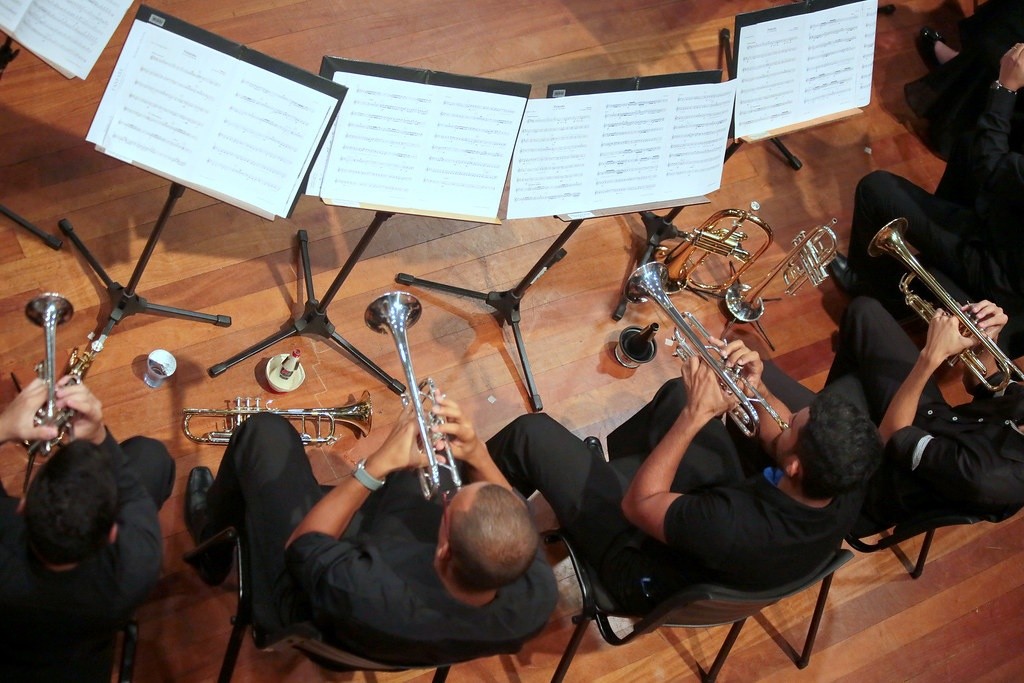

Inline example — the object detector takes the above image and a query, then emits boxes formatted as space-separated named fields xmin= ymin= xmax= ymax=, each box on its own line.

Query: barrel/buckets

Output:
xmin=265 ymin=354 xmax=306 ymax=393
xmin=614 ymin=326 xmax=658 ymax=369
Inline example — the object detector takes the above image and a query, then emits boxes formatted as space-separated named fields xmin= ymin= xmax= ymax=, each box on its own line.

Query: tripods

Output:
xmin=57 ymin=0 xmax=880 ymax=413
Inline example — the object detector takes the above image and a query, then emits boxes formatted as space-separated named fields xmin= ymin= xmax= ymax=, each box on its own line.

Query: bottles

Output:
xmin=624 ymin=322 xmax=659 ymax=360
xmin=277 ymin=348 xmax=301 ymax=384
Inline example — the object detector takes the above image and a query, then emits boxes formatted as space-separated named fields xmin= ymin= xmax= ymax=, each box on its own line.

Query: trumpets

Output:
xmin=725 ymin=216 xmax=840 ymax=322
xmin=867 ymin=217 xmax=1024 ymax=393
xmin=622 ymin=261 xmax=790 ymax=437
xmin=181 ymin=388 xmax=373 ymax=445
xmin=364 ymin=291 xmax=462 ymax=501
xmin=24 ymin=292 xmax=96 ymax=458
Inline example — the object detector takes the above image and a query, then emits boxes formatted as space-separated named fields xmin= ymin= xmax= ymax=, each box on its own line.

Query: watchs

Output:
xmin=350 ymin=458 xmax=386 ymax=491
xmin=989 ymin=80 xmax=1017 ymax=96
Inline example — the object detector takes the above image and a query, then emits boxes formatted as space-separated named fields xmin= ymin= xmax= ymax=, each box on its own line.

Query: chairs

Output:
xmin=0 ymin=506 xmax=1024 ymax=683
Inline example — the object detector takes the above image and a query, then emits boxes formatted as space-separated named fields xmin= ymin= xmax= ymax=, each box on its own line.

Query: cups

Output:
xmin=143 ymin=349 xmax=177 ymax=388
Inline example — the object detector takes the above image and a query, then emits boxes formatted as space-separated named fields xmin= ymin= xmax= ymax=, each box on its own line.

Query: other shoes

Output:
xmin=918 ymin=25 xmax=948 ymax=73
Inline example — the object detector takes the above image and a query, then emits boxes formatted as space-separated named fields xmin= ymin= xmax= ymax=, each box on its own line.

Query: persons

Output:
xmin=417 ymin=335 xmax=885 ymax=626
xmin=0 ymin=373 xmax=176 ymax=645
xmin=825 ymin=0 xmax=1024 ymax=338
xmin=184 ymin=393 xmax=559 ymax=673
xmin=726 ymin=296 xmax=1024 ymax=523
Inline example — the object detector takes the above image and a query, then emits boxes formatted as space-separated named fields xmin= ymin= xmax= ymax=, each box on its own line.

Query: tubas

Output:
xmin=658 ymin=200 xmax=774 ymax=296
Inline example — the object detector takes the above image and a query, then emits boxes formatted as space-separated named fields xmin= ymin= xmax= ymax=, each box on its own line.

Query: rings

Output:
xmin=411 ymin=409 xmax=415 ymax=418
xmin=1011 ymin=46 xmax=1017 ymax=50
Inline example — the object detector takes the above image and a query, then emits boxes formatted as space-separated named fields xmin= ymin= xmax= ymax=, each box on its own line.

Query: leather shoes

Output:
xmin=183 ymin=465 xmax=215 ymax=544
xmin=581 ymin=435 xmax=607 ymax=463
xmin=827 ymin=250 xmax=853 ymax=299
